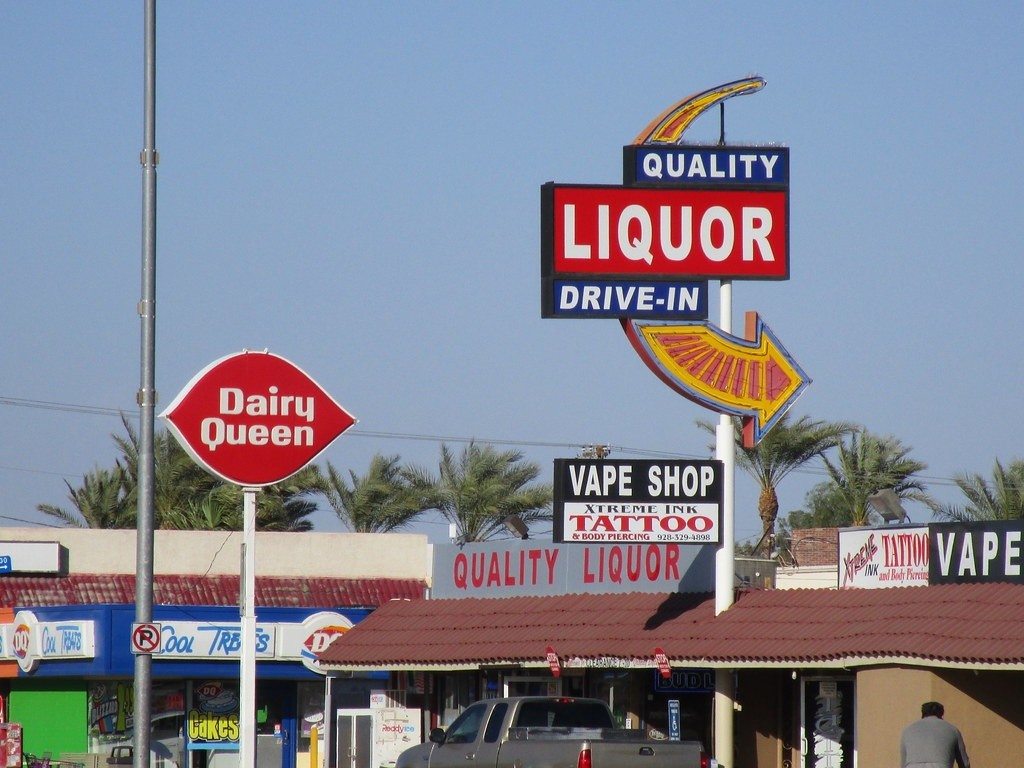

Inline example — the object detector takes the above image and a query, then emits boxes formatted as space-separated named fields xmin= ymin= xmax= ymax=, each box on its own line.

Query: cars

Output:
xmin=97 ymin=710 xmax=185 ymax=768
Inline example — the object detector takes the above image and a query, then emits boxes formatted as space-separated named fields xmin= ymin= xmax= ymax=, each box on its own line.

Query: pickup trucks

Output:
xmin=393 ymin=696 xmax=708 ymax=768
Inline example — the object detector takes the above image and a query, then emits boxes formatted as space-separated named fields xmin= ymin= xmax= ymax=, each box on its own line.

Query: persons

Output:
xmin=900 ymin=701 xmax=970 ymax=768
